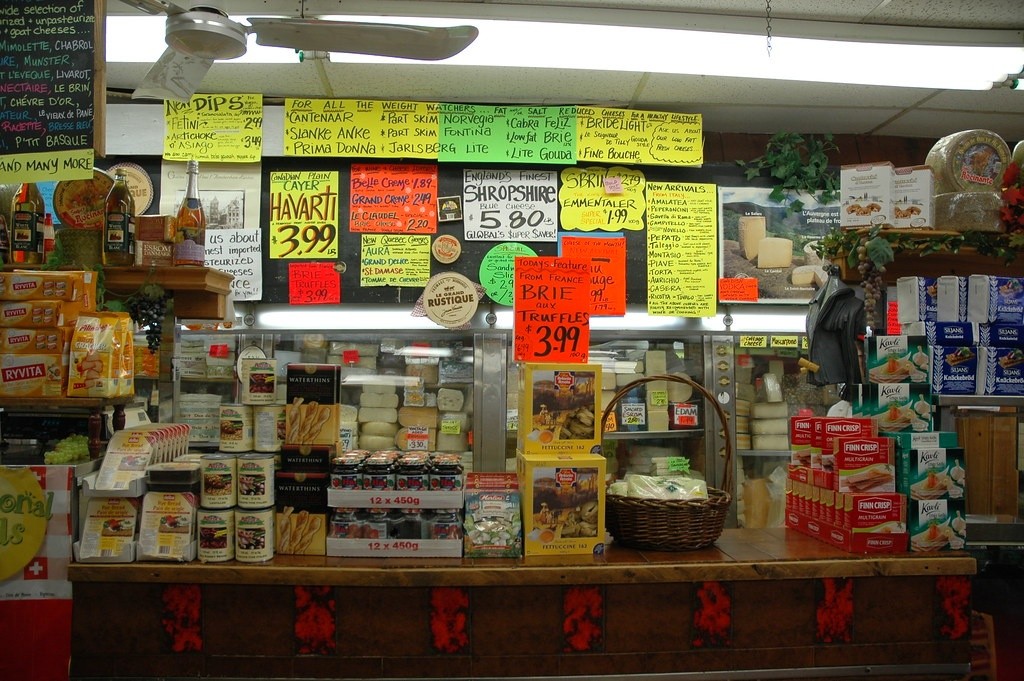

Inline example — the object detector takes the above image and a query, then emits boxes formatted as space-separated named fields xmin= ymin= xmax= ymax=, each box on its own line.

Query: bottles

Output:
xmin=43 ymin=213 xmax=55 ymax=264
xmin=10 ymin=183 xmax=45 ymax=264
xmin=102 ymin=168 xmax=135 ymax=267
xmin=174 ymin=160 xmax=206 ymax=267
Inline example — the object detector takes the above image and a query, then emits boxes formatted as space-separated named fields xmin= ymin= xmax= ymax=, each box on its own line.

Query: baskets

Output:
xmin=601 ymin=373 xmax=731 ymax=552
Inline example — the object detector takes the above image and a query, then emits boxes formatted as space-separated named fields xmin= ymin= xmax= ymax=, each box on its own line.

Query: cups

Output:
xmin=744 ymin=478 xmax=773 ymax=528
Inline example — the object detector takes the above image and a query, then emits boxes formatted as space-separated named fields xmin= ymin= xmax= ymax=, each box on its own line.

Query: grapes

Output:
xmin=128 ymin=288 xmax=174 ymax=355
xmin=43 ymin=433 xmax=91 ymax=463
xmin=857 ymin=239 xmax=885 ymax=330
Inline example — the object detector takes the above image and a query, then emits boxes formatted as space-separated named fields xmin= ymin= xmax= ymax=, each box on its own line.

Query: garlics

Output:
xmin=908 ymin=346 xmax=966 ymax=549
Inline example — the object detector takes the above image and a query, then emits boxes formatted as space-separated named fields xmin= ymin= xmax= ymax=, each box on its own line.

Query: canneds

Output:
xmin=198 ymin=359 xmax=287 ymax=562
xmin=331 ymin=450 xmax=463 ymax=540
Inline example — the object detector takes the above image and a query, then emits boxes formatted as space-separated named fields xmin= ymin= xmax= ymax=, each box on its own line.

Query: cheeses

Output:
xmin=180 ymin=332 xmax=470 ymax=456
xmin=588 ymin=351 xmax=710 ymax=504
xmin=734 ymin=374 xmax=790 ymax=529
xmin=739 ymin=216 xmax=835 ymax=288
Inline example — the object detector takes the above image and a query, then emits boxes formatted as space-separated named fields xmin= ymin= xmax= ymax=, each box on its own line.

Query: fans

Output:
xmin=119 ymin=0 xmax=480 ymax=104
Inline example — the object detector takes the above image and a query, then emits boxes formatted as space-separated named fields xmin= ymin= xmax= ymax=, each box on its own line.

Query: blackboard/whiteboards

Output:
xmin=0 ymin=0 xmax=108 ymax=161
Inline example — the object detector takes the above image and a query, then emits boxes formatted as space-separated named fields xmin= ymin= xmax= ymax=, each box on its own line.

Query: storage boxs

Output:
xmin=833 ymin=437 xmax=895 ymax=492
xmin=336 ymin=364 xmax=342 ymax=442
xmin=849 ymin=383 xmax=931 ymax=437
xmin=285 ymin=363 xmax=337 ymax=445
xmin=893 ymin=164 xmax=935 ymax=230
xmin=810 ymin=416 xmax=845 ymax=469
xmin=516 ymin=452 xmax=524 ymax=507
xmin=281 ymin=445 xmax=332 ymax=475
xmin=840 ymin=161 xmax=893 ymax=229
xmin=274 ymin=473 xmax=327 ymax=556
xmin=896 ymin=433 xmax=968 ymax=554
xmin=821 ymin=418 xmax=877 ymax=472
xmin=784 ymin=464 xmax=834 ymax=537
xmin=835 ymin=492 xmax=845 ymax=530
xmin=517 ymin=363 xmax=602 ymax=455
xmin=845 ymin=493 xmax=907 ymax=534
xmin=791 ymin=415 xmax=812 ymax=465
xmin=864 ymin=336 xmax=929 ymax=383
xmin=523 ymin=457 xmax=607 ymax=556
